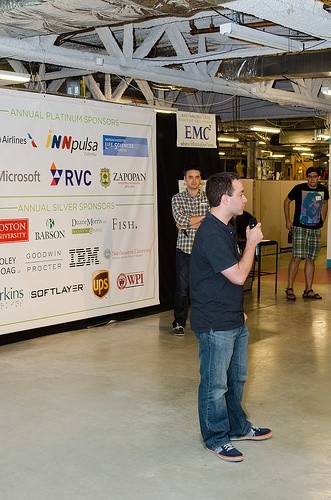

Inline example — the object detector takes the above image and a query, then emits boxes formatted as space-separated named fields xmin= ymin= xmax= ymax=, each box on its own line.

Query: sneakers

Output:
xmin=171 ymin=321 xmax=184 ymax=335
xmin=230 ymin=427 xmax=272 ymax=440
xmin=205 ymin=442 xmax=244 ymax=462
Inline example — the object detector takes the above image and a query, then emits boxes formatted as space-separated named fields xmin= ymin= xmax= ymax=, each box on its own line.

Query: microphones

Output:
xmin=249 ymin=218 xmax=260 ymax=262
xmin=180 ymin=229 xmax=188 ymax=236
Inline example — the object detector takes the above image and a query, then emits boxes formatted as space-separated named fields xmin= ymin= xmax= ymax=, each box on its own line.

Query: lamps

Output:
xmin=220 ymin=23 xmax=304 ymax=54
xmin=0 ymin=70 xmax=31 ymax=83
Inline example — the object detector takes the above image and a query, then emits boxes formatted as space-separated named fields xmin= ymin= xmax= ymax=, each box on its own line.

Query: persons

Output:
xmin=188 ymin=172 xmax=274 ymax=462
xmin=285 ymin=167 xmax=329 ymax=302
xmin=172 ymin=167 xmax=211 ymax=337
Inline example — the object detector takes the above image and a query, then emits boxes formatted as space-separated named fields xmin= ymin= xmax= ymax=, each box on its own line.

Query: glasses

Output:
xmin=307 ymin=175 xmax=318 ymax=178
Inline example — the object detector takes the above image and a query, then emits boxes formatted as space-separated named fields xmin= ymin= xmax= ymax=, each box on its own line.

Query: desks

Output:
xmin=239 ymin=240 xmax=280 ymax=298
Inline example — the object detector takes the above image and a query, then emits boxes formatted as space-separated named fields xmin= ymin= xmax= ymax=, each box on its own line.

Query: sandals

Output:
xmin=284 ymin=288 xmax=295 ymax=300
xmin=302 ymin=290 xmax=322 ymax=300
